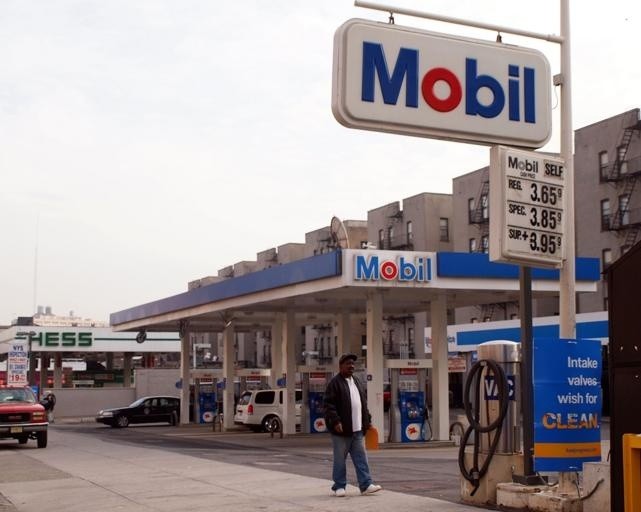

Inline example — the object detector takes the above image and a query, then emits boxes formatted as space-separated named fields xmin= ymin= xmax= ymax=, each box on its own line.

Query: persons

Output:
xmin=323 ymin=353 xmax=383 ymax=497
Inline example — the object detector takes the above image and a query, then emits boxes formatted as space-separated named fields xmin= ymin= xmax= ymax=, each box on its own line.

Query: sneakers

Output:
xmin=335 ymin=488 xmax=346 ymax=497
xmin=362 ymin=484 xmax=382 ymax=496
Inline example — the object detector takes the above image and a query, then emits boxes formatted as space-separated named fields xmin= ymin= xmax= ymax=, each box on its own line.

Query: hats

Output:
xmin=339 ymin=354 xmax=357 ymax=364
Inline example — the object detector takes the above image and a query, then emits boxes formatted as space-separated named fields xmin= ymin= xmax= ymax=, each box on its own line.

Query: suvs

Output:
xmin=383 ymin=381 xmax=391 ymax=410
xmin=0 ymin=386 xmax=48 ymax=448
xmin=234 ymin=388 xmax=303 ymax=432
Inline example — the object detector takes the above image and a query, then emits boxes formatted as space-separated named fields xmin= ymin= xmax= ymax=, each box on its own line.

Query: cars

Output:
xmin=449 ymin=404 xmax=474 ymax=444
xmin=96 ymin=396 xmax=180 ymax=428
